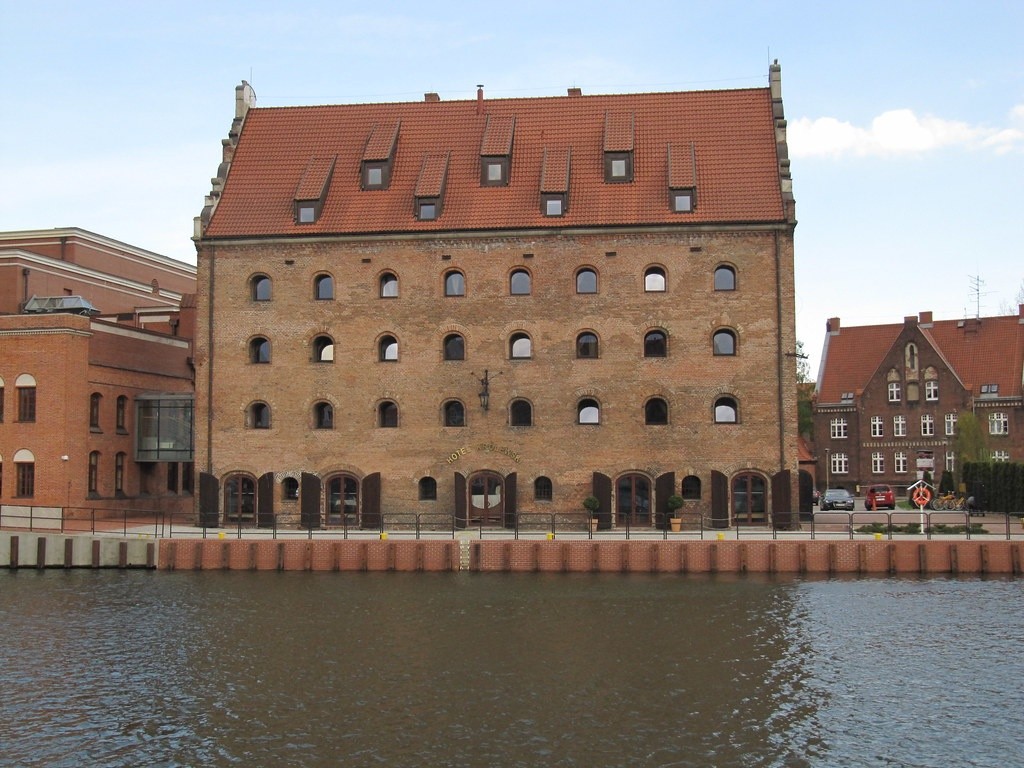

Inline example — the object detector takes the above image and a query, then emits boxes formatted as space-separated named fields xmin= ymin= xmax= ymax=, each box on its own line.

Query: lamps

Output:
xmin=470 ymin=369 xmax=503 ymax=410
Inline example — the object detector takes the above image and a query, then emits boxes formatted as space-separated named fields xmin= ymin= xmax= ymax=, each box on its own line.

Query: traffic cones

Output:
xmin=871 ymin=498 xmax=877 ymax=510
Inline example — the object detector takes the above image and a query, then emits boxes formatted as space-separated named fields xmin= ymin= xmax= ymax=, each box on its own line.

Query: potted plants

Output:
xmin=668 ymin=496 xmax=683 ymax=532
xmin=583 ymin=496 xmax=598 ymax=532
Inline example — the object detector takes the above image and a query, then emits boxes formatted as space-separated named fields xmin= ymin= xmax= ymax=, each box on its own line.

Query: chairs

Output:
xmin=967 ymin=481 xmax=987 ymax=517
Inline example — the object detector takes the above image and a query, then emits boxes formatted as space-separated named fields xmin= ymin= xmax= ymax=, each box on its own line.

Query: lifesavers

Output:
xmin=913 ymin=487 xmax=931 ymax=505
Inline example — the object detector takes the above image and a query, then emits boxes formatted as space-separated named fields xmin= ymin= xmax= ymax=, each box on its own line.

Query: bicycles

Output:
xmin=930 ymin=489 xmax=967 ymax=510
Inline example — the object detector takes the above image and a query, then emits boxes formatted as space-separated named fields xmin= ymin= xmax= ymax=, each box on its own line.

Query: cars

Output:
xmin=864 ymin=484 xmax=896 ymax=511
xmin=813 ymin=488 xmax=855 ymax=511
